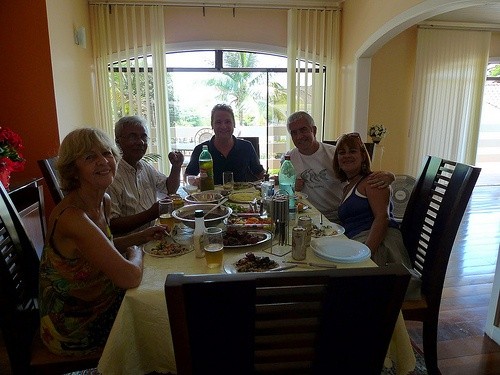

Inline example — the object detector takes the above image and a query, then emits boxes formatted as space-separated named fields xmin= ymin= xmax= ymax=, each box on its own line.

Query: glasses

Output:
xmin=117 ymin=135 xmax=150 ymax=142
xmin=337 ymin=132 xmax=362 ymax=145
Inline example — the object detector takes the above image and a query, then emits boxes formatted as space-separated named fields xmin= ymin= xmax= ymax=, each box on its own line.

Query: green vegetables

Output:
xmin=223 ymin=203 xmax=248 ymax=212
xmin=311 ymin=224 xmax=329 ymax=235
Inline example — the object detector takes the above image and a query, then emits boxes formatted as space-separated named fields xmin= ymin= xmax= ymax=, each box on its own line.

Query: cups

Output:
xmin=158 ymin=198 xmax=175 ymax=227
xmin=187 ymin=185 xmax=198 ymax=194
xmin=169 ymin=194 xmax=180 ymax=200
xmin=203 ymin=227 xmax=224 ymax=268
xmin=223 ymin=172 xmax=233 ymax=194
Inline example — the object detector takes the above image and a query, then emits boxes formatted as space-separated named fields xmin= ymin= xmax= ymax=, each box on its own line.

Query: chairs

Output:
xmin=0 ymin=137 xmax=482 ymax=375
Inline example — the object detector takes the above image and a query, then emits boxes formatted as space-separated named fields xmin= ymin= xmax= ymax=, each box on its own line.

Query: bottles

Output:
xmin=172 ymin=197 xmax=184 ymax=210
xmin=278 ymin=155 xmax=296 ymax=209
xmin=194 ymin=209 xmax=207 ymax=258
xmin=261 ymin=174 xmax=274 ymax=197
xmin=198 ymin=145 xmax=214 ymax=191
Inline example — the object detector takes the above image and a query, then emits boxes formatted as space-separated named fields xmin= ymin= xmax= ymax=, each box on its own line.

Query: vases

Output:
xmin=372 ymin=136 xmax=384 ymax=144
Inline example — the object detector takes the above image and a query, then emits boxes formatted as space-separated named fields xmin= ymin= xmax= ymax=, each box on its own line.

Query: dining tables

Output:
xmin=98 ymin=183 xmax=417 ymax=375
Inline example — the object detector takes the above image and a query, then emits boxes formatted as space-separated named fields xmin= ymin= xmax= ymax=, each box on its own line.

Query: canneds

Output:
xmin=168 ymin=193 xmax=184 ymax=223
xmin=297 ymin=216 xmax=312 ymax=247
xmin=291 ymin=225 xmax=306 ymax=260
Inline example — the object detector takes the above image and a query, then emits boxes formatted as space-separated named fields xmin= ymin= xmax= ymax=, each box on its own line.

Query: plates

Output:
xmin=142 ymin=181 xmax=309 ymax=257
xmin=223 ymin=252 xmax=298 ymax=274
xmin=309 ymin=221 xmax=371 ymax=263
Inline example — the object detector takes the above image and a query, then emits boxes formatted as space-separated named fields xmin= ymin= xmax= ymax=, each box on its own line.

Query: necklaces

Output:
xmin=346 ymin=172 xmax=361 ymax=181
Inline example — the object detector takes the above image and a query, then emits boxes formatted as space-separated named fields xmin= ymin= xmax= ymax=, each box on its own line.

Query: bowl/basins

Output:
xmin=183 ymin=192 xmax=224 ymax=205
xmin=171 ymin=203 xmax=233 ymax=230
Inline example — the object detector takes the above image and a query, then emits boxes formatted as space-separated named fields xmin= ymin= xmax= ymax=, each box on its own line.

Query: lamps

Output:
xmin=75 ymin=26 xmax=86 ymax=48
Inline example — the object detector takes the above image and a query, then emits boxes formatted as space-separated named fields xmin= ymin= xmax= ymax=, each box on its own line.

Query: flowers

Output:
xmin=0 ymin=125 xmax=26 ymax=191
xmin=369 ymin=124 xmax=389 ymax=137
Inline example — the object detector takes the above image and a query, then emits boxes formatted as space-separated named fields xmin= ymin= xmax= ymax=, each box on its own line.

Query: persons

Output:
xmin=280 ymin=111 xmax=395 ymax=228
xmin=105 ymin=116 xmax=184 ymax=234
xmin=184 ymin=104 xmax=265 ymax=188
xmin=40 ymin=129 xmax=170 ymax=360
xmin=333 ymin=133 xmax=411 ymax=275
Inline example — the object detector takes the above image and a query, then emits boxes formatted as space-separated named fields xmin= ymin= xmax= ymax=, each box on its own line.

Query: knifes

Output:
xmin=282 ymin=260 xmax=337 ymax=268
